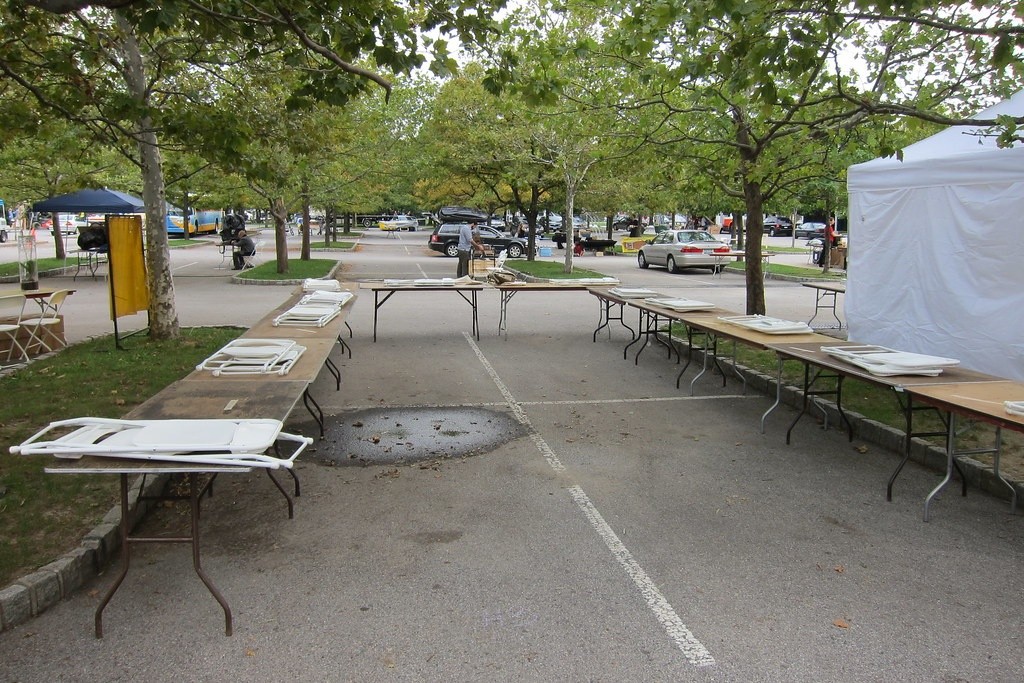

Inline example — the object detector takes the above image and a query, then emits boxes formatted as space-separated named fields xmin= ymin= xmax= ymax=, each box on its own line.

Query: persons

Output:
xmin=8 ymin=205 xmax=19 ymax=225
xmin=575 ymin=230 xmax=579 ymax=240
xmin=457 ymin=220 xmax=484 ymax=277
xmin=702 ymin=217 xmax=707 ymax=230
xmin=231 ymin=230 xmax=255 ymax=270
xmin=317 ymin=221 xmax=325 ymax=235
xmin=26 ymin=206 xmax=31 ymax=219
xmin=79 ymin=212 xmax=85 ymax=222
xmin=819 ymin=217 xmax=842 ymax=267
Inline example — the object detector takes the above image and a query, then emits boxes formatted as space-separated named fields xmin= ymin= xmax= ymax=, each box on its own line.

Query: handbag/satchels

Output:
xmin=488 ymin=271 xmax=516 ymax=281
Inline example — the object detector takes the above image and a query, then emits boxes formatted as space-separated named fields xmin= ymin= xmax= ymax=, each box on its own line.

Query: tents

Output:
xmin=29 ymin=190 xmax=146 ymax=274
xmin=844 ymin=88 xmax=1024 ymax=389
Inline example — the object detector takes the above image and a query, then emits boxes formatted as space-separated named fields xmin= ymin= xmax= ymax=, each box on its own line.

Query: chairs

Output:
xmin=272 ymin=279 xmax=457 ymax=328
xmin=17 ymin=289 xmax=70 ymax=359
xmin=7 ymin=415 xmax=313 ymax=471
xmin=196 ymin=336 xmax=297 ymax=373
xmin=239 ymin=240 xmax=266 ymax=271
xmin=545 ymin=276 xmax=962 ymax=377
xmin=211 ymin=344 xmax=307 ymax=376
xmin=0 ymin=293 xmax=31 ymax=364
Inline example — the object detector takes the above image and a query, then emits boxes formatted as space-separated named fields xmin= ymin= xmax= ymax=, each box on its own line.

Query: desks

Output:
xmin=359 ymin=281 xmax=484 ymax=342
xmin=678 ymin=314 xmax=848 ymax=434
xmin=706 ymin=254 xmax=776 ymax=282
xmin=45 ymin=379 xmax=327 ymax=640
xmin=805 ymin=244 xmax=824 ymax=264
xmin=886 ymin=381 xmax=1024 ymax=523
xmin=801 ymin=282 xmax=846 ymax=331
xmin=216 ymin=243 xmax=242 ymax=269
xmin=495 ymin=276 xmax=619 ymax=338
xmin=635 ymin=303 xmax=746 ymax=389
xmin=587 ymin=286 xmax=673 ymax=343
xmin=253 ymin=294 xmax=352 ymax=385
xmin=763 ymin=340 xmax=1014 ymax=502
xmin=184 ymin=336 xmax=340 ymax=440
xmin=0 ymin=286 xmax=78 ymax=345
xmin=72 ymin=249 xmax=109 ymax=283
xmin=470 ymin=244 xmax=496 ymax=273
xmin=291 ymin=280 xmax=358 ymax=354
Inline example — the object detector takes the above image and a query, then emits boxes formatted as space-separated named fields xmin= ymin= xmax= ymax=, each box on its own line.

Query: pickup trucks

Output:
xmin=356 ymin=210 xmax=388 ymax=228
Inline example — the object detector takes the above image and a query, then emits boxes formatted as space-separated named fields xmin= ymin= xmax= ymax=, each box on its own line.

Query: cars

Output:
xmin=392 ymin=215 xmax=418 ymax=232
xmin=763 ymin=217 xmax=794 ymax=237
xmin=613 ymin=216 xmax=648 ymax=232
xmin=49 ymin=215 xmax=91 ymax=237
xmin=486 ymin=212 xmax=588 ymax=239
xmin=792 ymin=222 xmax=825 ymax=240
xmin=638 ymin=230 xmax=732 ymax=274
xmin=0 ymin=200 xmax=12 ymax=243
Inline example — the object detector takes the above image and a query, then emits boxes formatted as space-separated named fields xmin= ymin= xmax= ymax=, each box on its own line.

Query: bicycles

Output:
xmin=284 ymin=222 xmax=294 ymax=236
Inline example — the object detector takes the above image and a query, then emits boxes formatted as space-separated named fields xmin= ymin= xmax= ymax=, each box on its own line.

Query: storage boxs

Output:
xmin=0 ymin=313 xmax=66 ymax=362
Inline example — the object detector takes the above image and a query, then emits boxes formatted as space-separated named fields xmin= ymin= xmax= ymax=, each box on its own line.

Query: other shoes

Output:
xmin=231 ymin=265 xmax=242 ymax=270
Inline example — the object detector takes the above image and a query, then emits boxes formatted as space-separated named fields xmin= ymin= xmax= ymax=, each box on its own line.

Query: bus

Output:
xmin=166 ymin=194 xmax=221 ymax=237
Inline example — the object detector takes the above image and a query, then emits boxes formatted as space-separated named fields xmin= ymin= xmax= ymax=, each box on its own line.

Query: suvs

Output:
xmin=428 ymin=207 xmax=538 ymax=259
